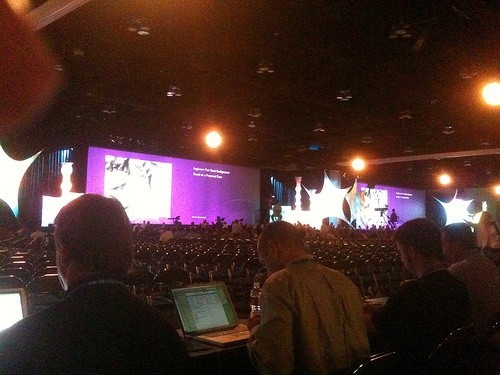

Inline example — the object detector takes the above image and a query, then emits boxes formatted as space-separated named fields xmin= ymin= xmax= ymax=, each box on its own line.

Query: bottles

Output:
xmin=250 ymin=282 xmax=262 ymax=318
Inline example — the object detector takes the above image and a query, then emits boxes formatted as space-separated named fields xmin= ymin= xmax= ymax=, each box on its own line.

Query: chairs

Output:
xmin=0 ymin=234 xmax=450 ymax=318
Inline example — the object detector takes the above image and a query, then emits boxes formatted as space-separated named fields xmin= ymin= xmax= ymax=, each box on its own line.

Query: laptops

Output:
xmin=0 ymin=288 xmax=28 ymax=332
xmin=170 ymin=280 xmax=251 ymax=348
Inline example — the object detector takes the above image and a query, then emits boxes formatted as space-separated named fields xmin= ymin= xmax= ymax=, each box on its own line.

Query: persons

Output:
xmin=363 ymin=218 xmax=475 ymax=375
xmin=400 ymin=223 xmax=500 ymax=350
xmin=0 ymin=193 xmax=192 ymax=375
xmin=0 ymin=200 xmax=500 ymax=250
xmin=246 ymin=220 xmax=372 ymax=375
